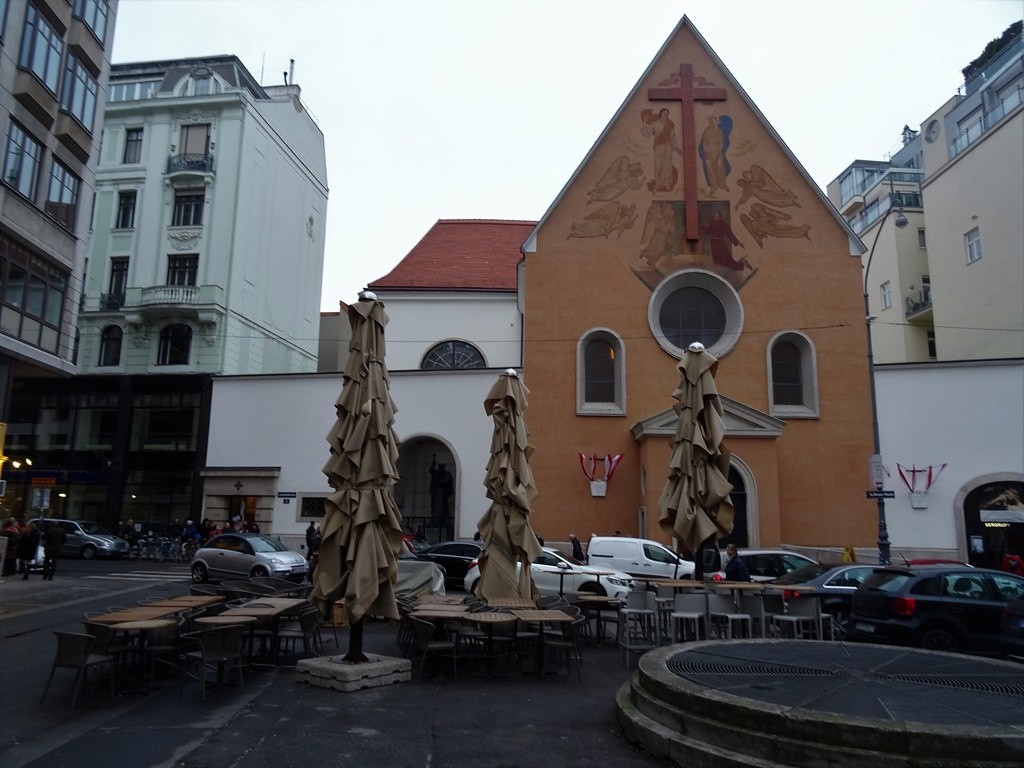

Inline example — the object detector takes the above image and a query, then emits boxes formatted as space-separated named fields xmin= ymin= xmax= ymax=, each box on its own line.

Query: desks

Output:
xmin=110 ymin=619 xmax=175 ymax=697
xmin=463 ymin=611 xmax=518 ymax=665
xmin=218 ymin=608 xmax=285 ymax=668
xmin=411 ymin=610 xmax=470 ymax=663
xmin=487 ymin=596 xmax=537 ymax=609
xmin=414 ymin=594 xmax=466 ymax=605
xmin=545 ymin=570 xmax=619 ymax=648
xmin=511 ymin=610 xmax=575 ymax=679
xmin=170 ymin=596 xmax=226 ymax=604
xmin=236 ymin=597 xmax=307 ymax=666
xmin=624 ymin=577 xmax=816 ymax=641
xmin=194 ymin=616 xmax=258 ymax=687
xmin=88 ymin=607 xmax=190 ymax=669
xmin=414 ymin=605 xmax=470 ymax=611
xmin=144 ymin=601 xmax=208 ymax=608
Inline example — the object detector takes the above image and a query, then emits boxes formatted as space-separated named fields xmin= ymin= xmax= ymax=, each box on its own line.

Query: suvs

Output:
xmin=25 ymin=519 xmax=130 ymax=560
xmin=701 ymin=546 xmax=819 ymax=586
xmin=848 ymin=566 xmax=1024 ymax=662
xmin=461 ymin=546 xmax=637 ymax=610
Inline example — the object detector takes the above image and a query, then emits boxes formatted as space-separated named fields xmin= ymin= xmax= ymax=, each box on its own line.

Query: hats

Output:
xmin=187 ymin=520 xmax=194 ymax=525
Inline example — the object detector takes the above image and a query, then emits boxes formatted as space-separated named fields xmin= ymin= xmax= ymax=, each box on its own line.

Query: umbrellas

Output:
xmin=474 ymin=368 xmax=542 ymax=599
xmin=658 ymin=342 xmax=735 ymax=590
xmin=308 ymin=292 xmax=404 ymax=663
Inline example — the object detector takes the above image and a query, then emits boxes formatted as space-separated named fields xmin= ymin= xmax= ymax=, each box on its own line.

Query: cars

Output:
xmin=190 ymin=529 xmax=309 ymax=587
xmin=310 ymin=534 xmax=431 ymax=568
xmin=771 ymin=561 xmax=885 ymax=641
xmin=900 ymin=552 xmax=973 ymax=568
xmin=414 ymin=539 xmax=485 ymax=580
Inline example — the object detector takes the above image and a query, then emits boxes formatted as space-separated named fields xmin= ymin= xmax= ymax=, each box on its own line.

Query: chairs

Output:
xmin=847 ymin=571 xmax=860 ymax=586
xmin=952 ymin=578 xmax=972 ymax=598
xmin=394 ymin=584 xmax=835 ymax=684
xmin=43 ymin=577 xmax=333 ymax=708
xmin=943 ymin=577 xmax=949 ymax=595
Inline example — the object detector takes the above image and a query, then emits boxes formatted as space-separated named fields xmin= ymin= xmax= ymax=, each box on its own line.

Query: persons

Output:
xmin=306 ymin=521 xmax=320 ymax=561
xmin=613 ymin=531 xmax=621 ymax=537
xmin=0 ymin=517 xmax=68 ymax=580
xmin=726 ymin=544 xmax=750 ymax=594
xmin=429 ymin=458 xmax=453 ymax=527
xmin=535 ymin=532 xmax=544 ymax=547
xmin=122 ymin=519 xmax=136 ymax=547
xmin=569 ymin=534 xmax=585 ymax=561
xmin=169 ymin=518 xmax=260 ymax=542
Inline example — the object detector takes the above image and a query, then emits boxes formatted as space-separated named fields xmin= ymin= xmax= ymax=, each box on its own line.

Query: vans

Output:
xmin=585 ymin=533 xmax=696 ymax=582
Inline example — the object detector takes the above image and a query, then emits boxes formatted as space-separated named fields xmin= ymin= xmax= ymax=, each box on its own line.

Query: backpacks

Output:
xmin=1005 ymin=556 xmax=1020 ymax=572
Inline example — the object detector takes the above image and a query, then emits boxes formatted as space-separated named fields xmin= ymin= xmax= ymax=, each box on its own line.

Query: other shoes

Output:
xmin=1 ymin=572 xmax=16 ymax=577
xmin=22 ymin=577 xmax=29 ymax=580
xmin=16 ymin=571 xmax=22 ymax=574
xmin=42 ymin=573 xmax=52 ymax=580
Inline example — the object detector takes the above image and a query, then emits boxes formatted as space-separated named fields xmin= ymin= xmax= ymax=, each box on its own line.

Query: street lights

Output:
xmin=11 ymin=458 xmax=32 ymax=519
